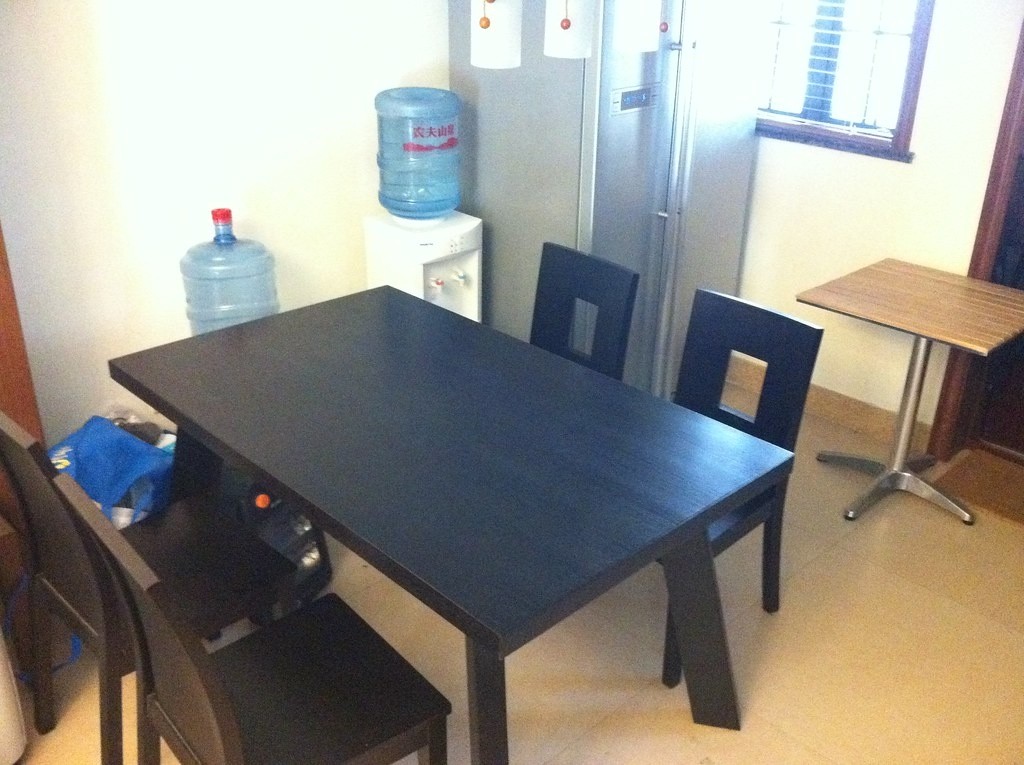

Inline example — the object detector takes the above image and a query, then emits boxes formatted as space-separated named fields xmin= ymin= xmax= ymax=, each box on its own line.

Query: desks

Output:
xmin=108 ymin=285 xmax=795 ymax=765
xmin=796 ymin=258 xmax=1024 ymax=525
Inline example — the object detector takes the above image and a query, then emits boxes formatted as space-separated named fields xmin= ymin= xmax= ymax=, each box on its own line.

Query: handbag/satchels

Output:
xmin=230 ymin=485 xmax=332 ymax=628
xmin=47 ymin=413 xmax=174 ymax=528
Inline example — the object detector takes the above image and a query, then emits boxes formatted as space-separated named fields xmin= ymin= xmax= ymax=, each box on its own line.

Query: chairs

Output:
xmin=0 ymin=412 xmax=451 ymax=765
xmin=530 ymin=241 xmax=640 ymax=383
xmin=655 ymin=288 xmax=826 ymax=689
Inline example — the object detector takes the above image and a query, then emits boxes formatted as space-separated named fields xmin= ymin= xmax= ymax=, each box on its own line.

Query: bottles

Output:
xmin=221 ymin=462 xmax=272 ymax=511
xmin=180 ymin=208 xmax=282 ymax=336
xmin=374 ymin=86 xmax=461 ymax=220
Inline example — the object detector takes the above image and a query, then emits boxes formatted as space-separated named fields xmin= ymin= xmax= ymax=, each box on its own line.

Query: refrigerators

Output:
xmin=449 ymin=1 xmax=765 ymax=401
